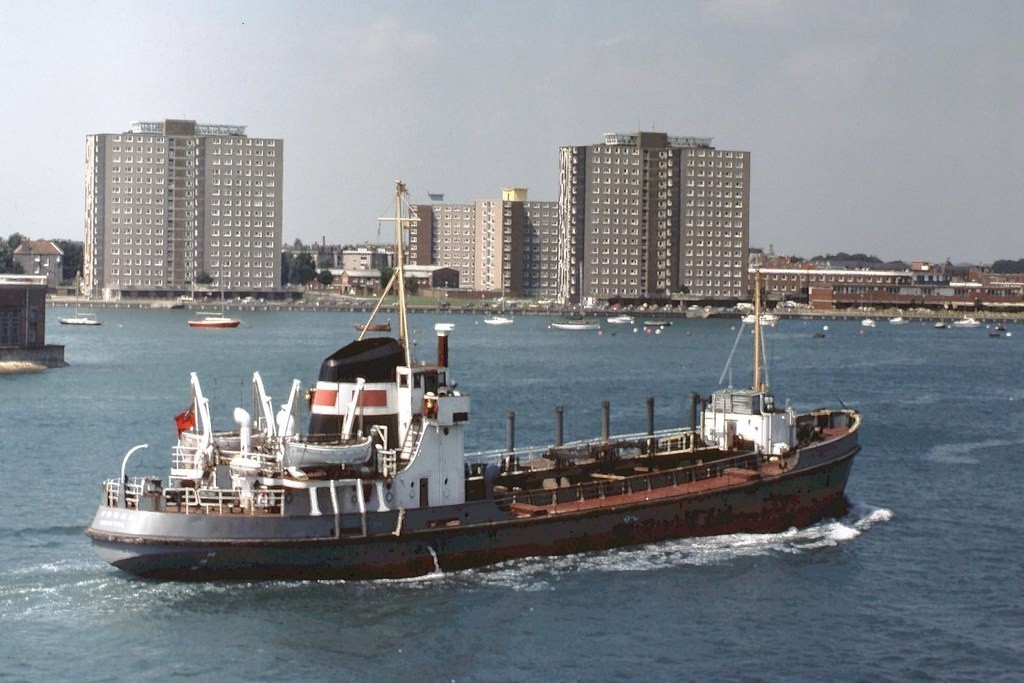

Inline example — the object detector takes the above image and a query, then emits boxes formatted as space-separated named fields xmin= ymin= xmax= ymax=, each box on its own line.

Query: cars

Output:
xmin=229 ymin=296 xmax=305 ymax=304
xmin=439 ymin=300 xmax=752 ymax=313
xmin=313 ymin=299 xmax=401 ymax=306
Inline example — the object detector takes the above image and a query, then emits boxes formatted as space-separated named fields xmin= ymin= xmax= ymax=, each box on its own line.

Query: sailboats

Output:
xmin=55 ymin=273 xmax=101 ymax=325
xmin=552 ymin=261 xmax=602 ymax=331
xmin=483 ymin=273 xmax=513 ymax=324
xmin=187 ymin=262 xmax=241 ymax=328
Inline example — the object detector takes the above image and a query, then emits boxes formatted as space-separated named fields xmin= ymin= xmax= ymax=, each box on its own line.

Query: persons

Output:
xmin=423 ymin=392 xmax=439 ymax=420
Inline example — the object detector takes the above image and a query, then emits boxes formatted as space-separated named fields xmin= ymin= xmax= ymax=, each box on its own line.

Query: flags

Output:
xmin=173 ymin=401 xmax=196 ymax=439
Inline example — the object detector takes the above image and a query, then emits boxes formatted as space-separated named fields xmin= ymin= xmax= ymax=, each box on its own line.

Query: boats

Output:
xmin=935 ymin=322 xmax=945 ymax=328
xmin=645 ymin=321 xmax=673 ymax=326
xmin=953 ymin=317 xmax=981 ymax=328
xmin=861 ymin=318 xmax=875 ymax=327
xmin=85 ymin=181 xmax=861 ymax=581
xmin=606 ymin=314 xmax=635 ymax=324
xmin=169 ymin=303 xmax=183 ymax=309
xmin=355 ymin=321 xmax=391 ymax=331
xmin=741 ymin=313 xmax=780 ymax=326
xmin=890 ymin=318 xmax=908 ymax=325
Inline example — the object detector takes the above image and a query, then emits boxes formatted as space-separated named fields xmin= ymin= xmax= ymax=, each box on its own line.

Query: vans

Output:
xmin=737 ymin=303 xmax=755 ymax=310
